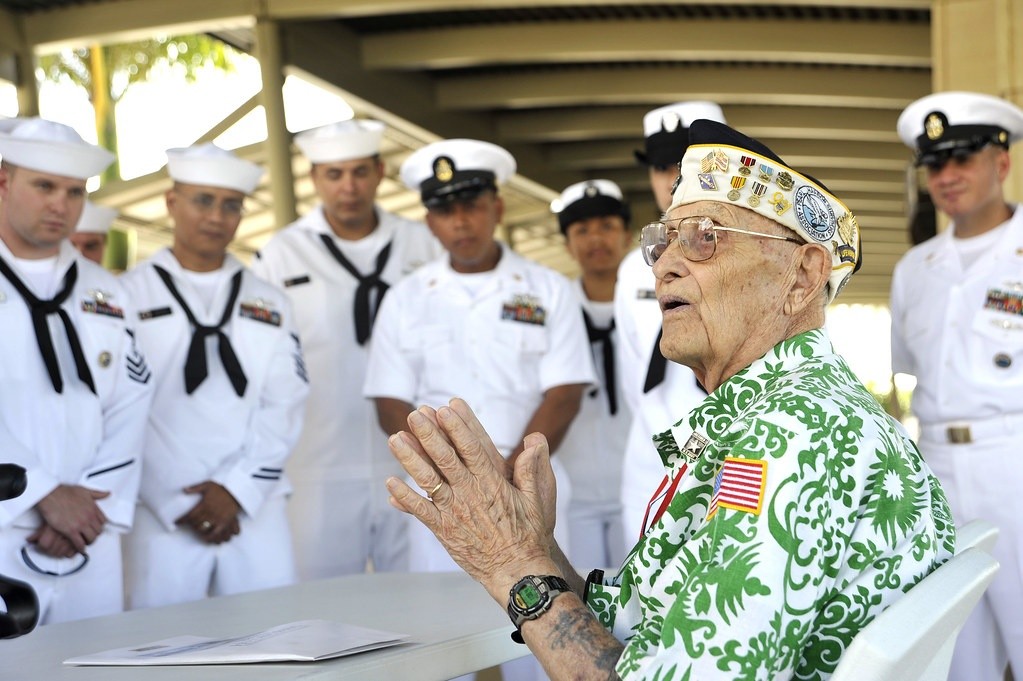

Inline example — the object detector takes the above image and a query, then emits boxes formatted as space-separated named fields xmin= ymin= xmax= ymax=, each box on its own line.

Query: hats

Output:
xmin=546 ymin=177 xmax=630 ymax=235
xmin=665 ymin=119 xmax=864 ymax=304
xmin=896 ymin=91 xmax=1023 ymax=167
xmin=0 ymin=116 xmax=116 ymax=181
xmin=75 ymin=199 xmax=117 ymax=233
xmin=399 ymin=139 xmax=515 ymax=211
xmin=166 ymin=142 xmax=264 ymax=196
xmin=633 ymin=100 xmax=726 ymax=165
xmin=294 ymin=119 xmax=385 ymax=163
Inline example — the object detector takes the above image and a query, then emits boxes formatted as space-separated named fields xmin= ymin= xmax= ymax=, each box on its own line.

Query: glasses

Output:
xmin=637 ymin=216 xmax=805 ymax=266
xmin=927 ymin=151 xmax=974 ymax=170
xmin=21 ymin=543 xmax=88 ymax=576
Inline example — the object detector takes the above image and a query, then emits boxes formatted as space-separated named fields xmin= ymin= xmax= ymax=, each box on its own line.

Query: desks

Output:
xmin=0 ymin=572 xmax=536 ymax=681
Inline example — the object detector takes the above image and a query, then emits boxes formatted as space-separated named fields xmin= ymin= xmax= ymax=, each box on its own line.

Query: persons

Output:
xmin=0 ymin=118 xmax=156 ymax=631
xmin=889 ymin=93 xmax=1023 ymax=681
xmin=550 ymin=99 xmax=728 ymax=571
xmin=115 ymin=142 xmax=310 ymax=612
xmin=251 ymin=118 xmax=447 ymax=579
xmin=362 ymin=138 xmax=600 ymax=574
xmin=385 ymin=119 xmax=956 ymax=681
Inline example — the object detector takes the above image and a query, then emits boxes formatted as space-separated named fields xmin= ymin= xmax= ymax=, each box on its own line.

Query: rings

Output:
xmin=428 ymin=477 xmax=443 ymax=498
xmin=202 ymin=521 xmax=211 ymax=529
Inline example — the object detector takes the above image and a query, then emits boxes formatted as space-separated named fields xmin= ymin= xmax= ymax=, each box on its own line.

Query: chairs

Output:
xmin=813 ymin=522 xmax=1002 ymax=681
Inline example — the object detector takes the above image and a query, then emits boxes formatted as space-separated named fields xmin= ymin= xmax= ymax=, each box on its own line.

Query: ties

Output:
xmin=154 ymin=264 xmax=248 ymax=398
xmin=0 ymin=256 xmax=99 ymax=396
xmin=320 ymin=234 xmax=391 ymax=345
xmin=582 ymin=308 xmax=617 ymax=415
xmin=643 ymin=322 xmax=708 ymax=395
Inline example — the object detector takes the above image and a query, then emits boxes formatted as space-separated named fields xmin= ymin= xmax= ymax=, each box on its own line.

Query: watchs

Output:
xmin=508 ymin=575 xmax=573 ymax=644
